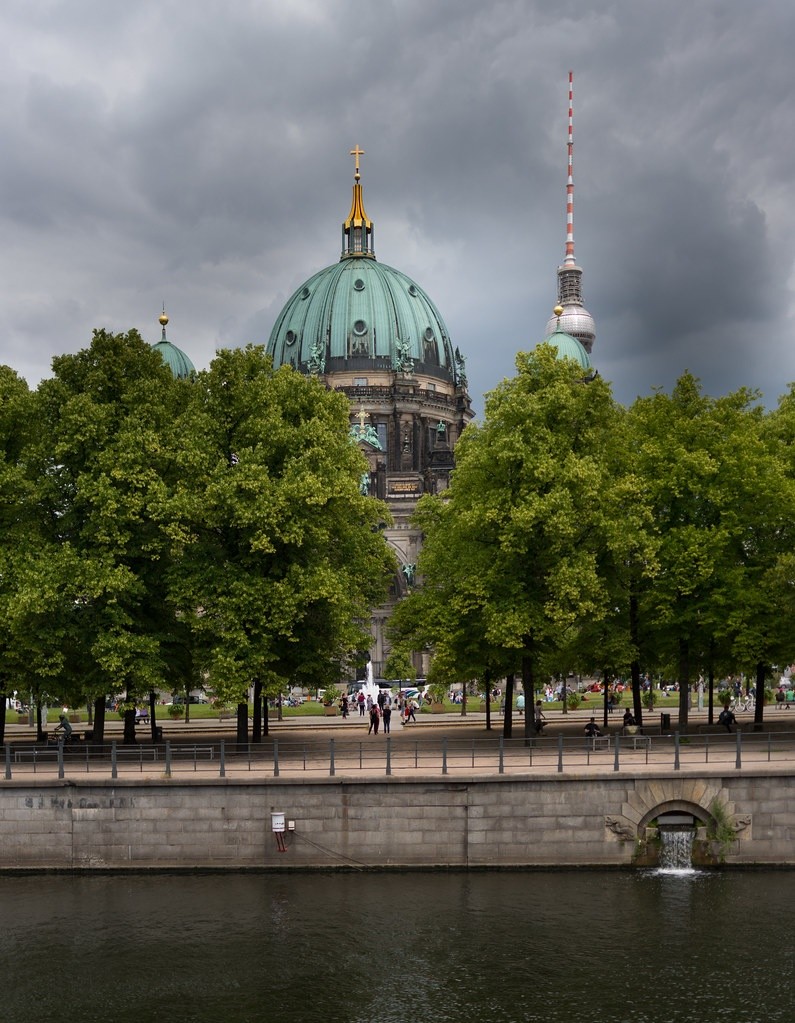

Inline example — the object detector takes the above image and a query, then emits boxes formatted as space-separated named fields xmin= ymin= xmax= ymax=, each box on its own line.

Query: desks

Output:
xmin=699 ymin=724 xmax=738 ymax=740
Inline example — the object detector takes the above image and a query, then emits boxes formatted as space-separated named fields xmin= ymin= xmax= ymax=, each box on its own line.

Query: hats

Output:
xmin=59 ymin=715 xmax=65 ymax=718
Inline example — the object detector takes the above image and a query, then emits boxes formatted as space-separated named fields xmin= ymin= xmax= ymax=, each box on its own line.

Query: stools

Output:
xmin=593 ymin=738 xmax=611 ymax=751
xmin=634 ymin=738 xmax=651 ymax=750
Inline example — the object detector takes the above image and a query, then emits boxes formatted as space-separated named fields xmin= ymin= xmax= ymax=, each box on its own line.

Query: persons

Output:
xmin=643 ymin=683 xmax=648 ymax=694
xmin=775 ymin=688 xmax=794 ymax=709
xmin=449 ymin=688 xmax=463 ymax=703
xmin=581 ymin=683 xmax=604 ymax=701
xmin=162 ymin=700 xmax=165 ymax=705
xmin=493 ymin=688 xmax=498 ymax=701
xmin=546 ymin=684 xmax=572 ymax=701
xmin=718 ymin=705 xmax=732 ymax=725
xmin=470 ymin=688 xmax=486 ymax=699
xmin=623 ymin=708 xmax=633 ymax=724
xmin=724 ymin=692 xmax=731 ymax=710
xmin=136 ymin=707 xmax=150 ymax=724
xmin=55 ymin=715 xmax=72 ymax=736
xmin=111 ymin=698 xmax=117 ymax=711
xmin=516 ymin=693 xmax=525 ymax=715
xmin=751 ymin=687 xmax=757 ymax=696
xmin=585 ymin=717 xmax=599 ymax=737
xmin=534 ymin=700 xmax=547 ymax=736
xmin=341 ymin=690 xmax=422 ymax=735
xmin=270 ymin=693 xmax=302 ymax=708
xmin=661 ymin=686 xmax=677 ymax=696
xmin=608 ymin=683 xmax=622 ymax=713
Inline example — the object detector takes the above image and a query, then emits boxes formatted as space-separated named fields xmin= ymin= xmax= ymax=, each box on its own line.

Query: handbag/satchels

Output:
xmin=405 ymin=707 xmax=409 ymax=716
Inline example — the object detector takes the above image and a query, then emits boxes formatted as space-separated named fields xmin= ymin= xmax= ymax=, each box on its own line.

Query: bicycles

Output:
xmin=48 ymin=728 xmax=82 ymax=753
xmin=727 ymin=691 xmax=756 ymax=713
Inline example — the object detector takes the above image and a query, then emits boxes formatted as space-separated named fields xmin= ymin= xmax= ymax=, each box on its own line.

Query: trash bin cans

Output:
xmin=151 ymin=727 xmax=163 ymax=742
xmin=661 ymin=713 xmax=670 ymax=729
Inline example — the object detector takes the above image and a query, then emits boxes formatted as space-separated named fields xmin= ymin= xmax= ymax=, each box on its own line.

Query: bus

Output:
xmin=354 ymin=678 xmax=431 ymax=700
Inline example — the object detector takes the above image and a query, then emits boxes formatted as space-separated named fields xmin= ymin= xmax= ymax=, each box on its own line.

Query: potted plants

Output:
xmin=646 ymin=821 xmax=659 ymax=840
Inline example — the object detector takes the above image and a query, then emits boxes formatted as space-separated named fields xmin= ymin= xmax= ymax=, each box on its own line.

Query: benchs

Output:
xmin=775 ymin=701 xmax=795 ymax=710
xmin=624 ymin=726 xmax=661 ymax=740
xmin=744 ymin=722 xmax=786 ymax=740
xmin=593 ymin=705 xmax=622 ymax=714
xmin=499 ymin=708 xmax=525 ymax=715
xmin=123 ymin=717 xmax=151 ymax=724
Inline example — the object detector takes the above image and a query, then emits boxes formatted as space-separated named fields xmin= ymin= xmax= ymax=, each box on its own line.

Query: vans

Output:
xmin=317 ymin=688 xmax=327 ymax=703
